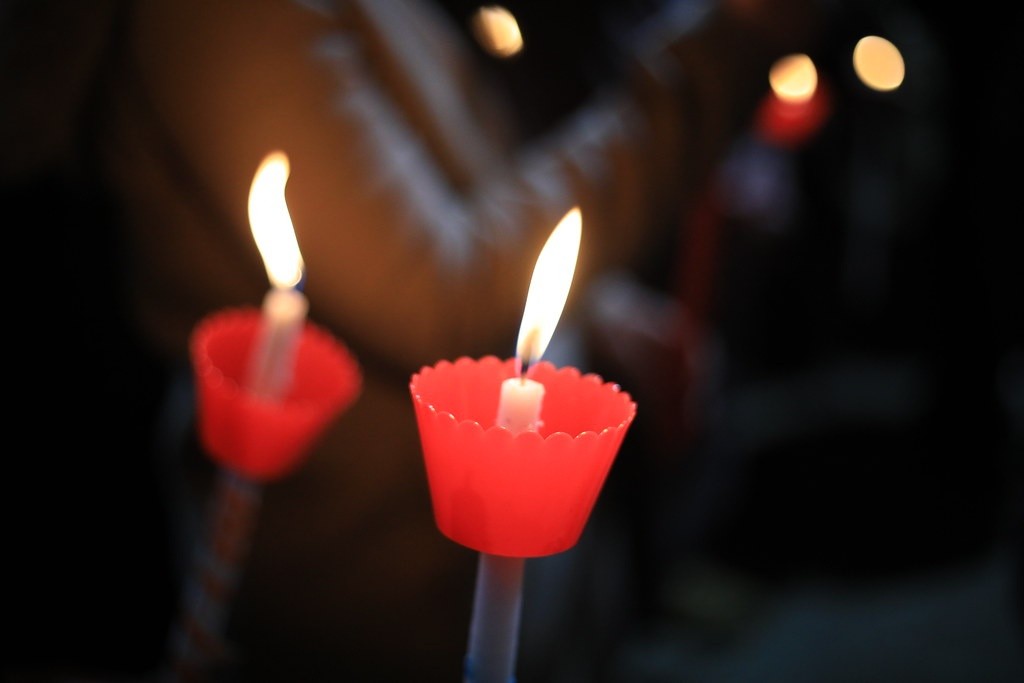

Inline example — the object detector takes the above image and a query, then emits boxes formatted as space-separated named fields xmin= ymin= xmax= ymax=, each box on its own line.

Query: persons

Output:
xmin=109 ymin=0 xmax=759 ymax=683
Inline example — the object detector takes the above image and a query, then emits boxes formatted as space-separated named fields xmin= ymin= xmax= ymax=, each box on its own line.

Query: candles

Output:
xmin=158 ymin=149 xmax=310 ymax=681
xmin=463 ymin=206 xmax=584 ymax=683
